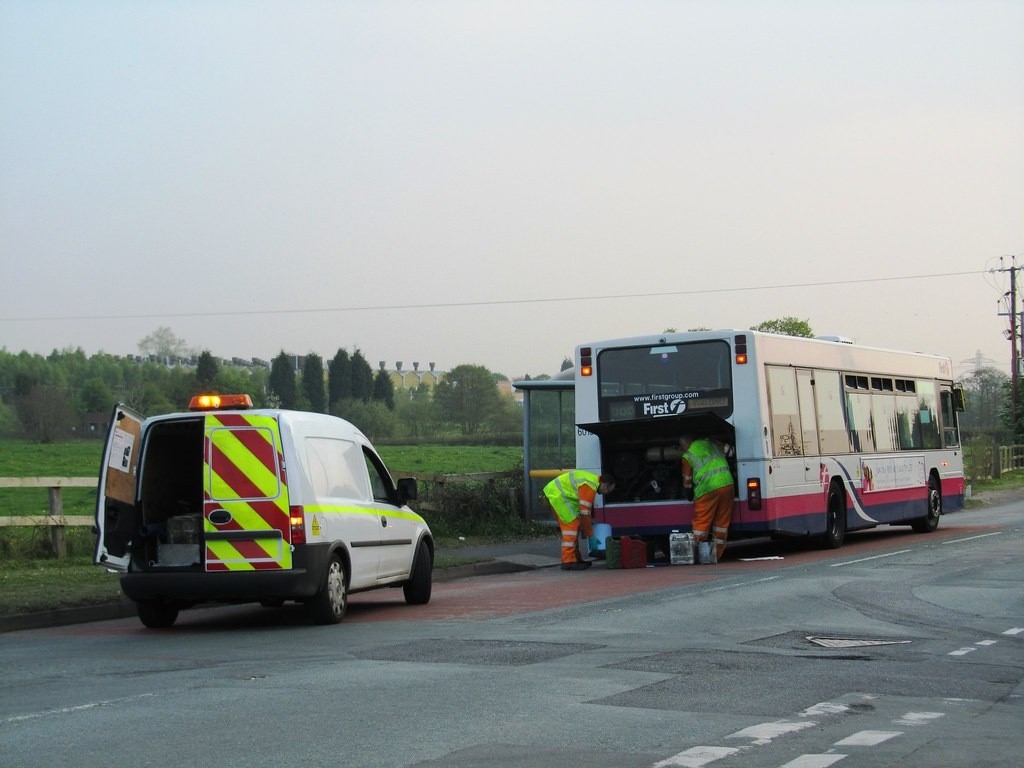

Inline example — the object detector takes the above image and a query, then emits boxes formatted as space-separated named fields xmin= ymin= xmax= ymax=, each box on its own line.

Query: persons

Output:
xmin=541 ymin=470 xmax=616 ymax=570
xmin=679 ymin=434 xmax=735 ymax=563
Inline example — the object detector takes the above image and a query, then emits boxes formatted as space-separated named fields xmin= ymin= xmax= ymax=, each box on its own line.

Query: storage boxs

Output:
xmin=167 ymin=512 xmax=203 ymax=545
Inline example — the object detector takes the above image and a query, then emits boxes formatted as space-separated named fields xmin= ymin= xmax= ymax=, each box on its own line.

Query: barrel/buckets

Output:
xmin=697 ymin=532 xmax=718 ymax=565
xmin=605 ymin=535 xmax=622 ymax=570
xmin=620 ymin=534 xmax=647 ymax=569
xmin=669 ymin=529 xmax=696 ymax=565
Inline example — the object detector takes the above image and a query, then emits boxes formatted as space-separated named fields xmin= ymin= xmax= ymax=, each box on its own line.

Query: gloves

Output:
xmin=588 ymin=536 xmax=601 ymax=553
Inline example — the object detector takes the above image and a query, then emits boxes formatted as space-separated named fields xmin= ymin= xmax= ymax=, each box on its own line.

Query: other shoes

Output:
xmin=561 ymin=558 xmax=592 ymax=570
